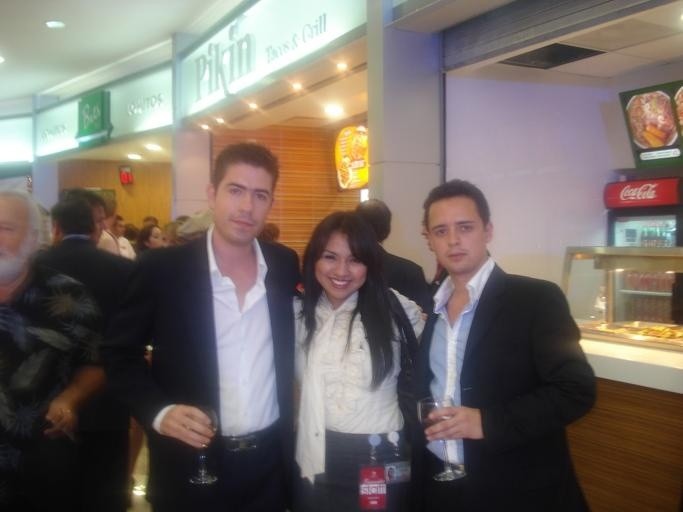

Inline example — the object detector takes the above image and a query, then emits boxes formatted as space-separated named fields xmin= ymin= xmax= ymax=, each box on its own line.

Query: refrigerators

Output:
xmin=603 ymin=177 xmax=683 ymax=324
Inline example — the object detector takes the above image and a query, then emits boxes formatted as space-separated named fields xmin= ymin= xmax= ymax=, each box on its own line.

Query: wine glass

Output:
xmin=418 ymin=393 xmax=470 ymax=482
xmin=184 ymin=408 xmax=218 ymax=485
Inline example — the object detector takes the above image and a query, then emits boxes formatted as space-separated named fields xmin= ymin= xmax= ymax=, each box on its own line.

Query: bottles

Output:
xmin=623 ymin=224 xmax=674 ymax=322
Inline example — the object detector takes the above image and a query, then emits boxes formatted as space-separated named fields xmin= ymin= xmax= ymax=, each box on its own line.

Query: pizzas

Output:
xmin=627 ymin=93 xmax=676 ymax=147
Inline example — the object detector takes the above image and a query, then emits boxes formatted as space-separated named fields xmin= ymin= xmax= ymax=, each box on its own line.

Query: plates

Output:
xmin=626 ymin=90 xmax=679 ymax=150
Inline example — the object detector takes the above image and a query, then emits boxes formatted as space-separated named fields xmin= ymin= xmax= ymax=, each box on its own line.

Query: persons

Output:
xmin=419 ymin=200 xmax=493 ymax=293
xmin=1 ymin=184 xmax=200 ymax=512
xmin=99 ymin=141 xmax=308 ymax=512
xmin=278 ymin=207 xmax=427 ymax=512
xmin=351 ymin=198 xmax=430 ymax=310
xmin=259 ymin=224 xmax=280 ymax=243
xmin=413 ymin=177 xmax=600 ymax=511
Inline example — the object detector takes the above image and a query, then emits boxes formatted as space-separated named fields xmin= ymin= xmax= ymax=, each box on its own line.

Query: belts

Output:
xmin=221 ymin=418 xmax=281 ymax=452
